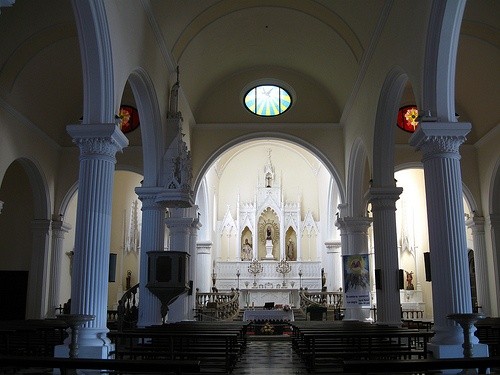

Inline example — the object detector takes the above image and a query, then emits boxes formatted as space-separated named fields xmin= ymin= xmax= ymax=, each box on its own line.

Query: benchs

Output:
xmin=288 ymin=320 xmax=435 ymax=375
xmin=1 ymin=319 xmax=248 ymax=375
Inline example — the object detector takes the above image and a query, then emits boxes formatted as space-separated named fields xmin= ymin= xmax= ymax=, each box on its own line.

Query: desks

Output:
xmin=243 ymin=309 xmax=295 ymax=322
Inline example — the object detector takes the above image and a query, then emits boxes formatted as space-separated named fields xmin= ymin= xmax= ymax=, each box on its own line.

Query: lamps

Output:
xmin=247 ymin=258 xmax=263 ymax=288
xmin=275 ymin=258 xmax=291 ymax=288
